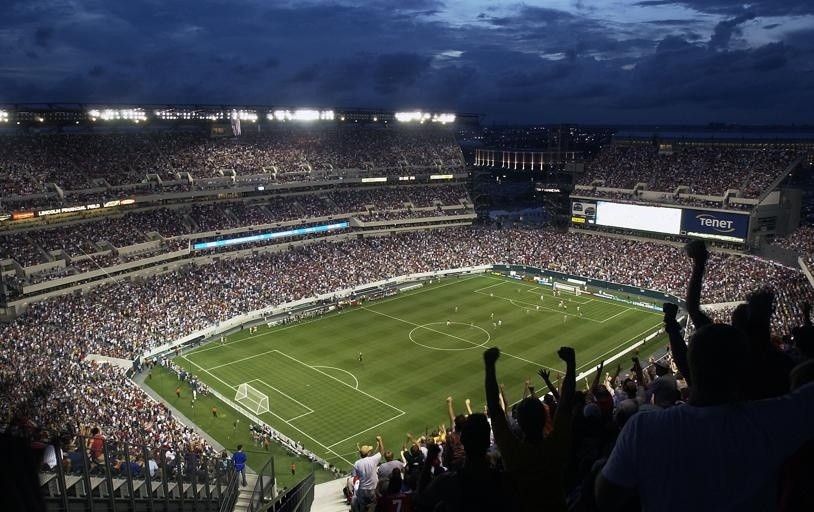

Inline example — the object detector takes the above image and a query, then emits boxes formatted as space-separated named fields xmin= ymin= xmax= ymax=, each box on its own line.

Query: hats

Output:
xmin=360 ymin=445 xmax=374 ymax=457
